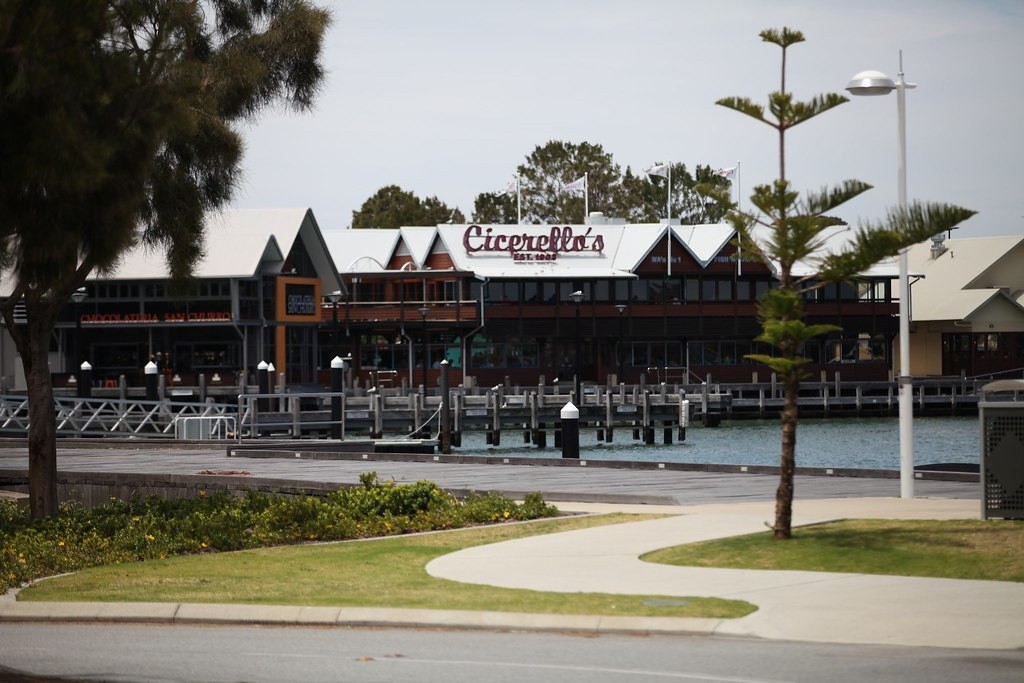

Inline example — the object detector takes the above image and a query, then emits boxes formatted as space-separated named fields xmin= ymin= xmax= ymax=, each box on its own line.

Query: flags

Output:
xmin=495 ymin=179 xmax=518 ymax=197
xmin=645 ymin=164 xmax=667 ymax=177
xmin=713 ymin=166 xmax=737 ymax=180
xmin=564 ymin=176 xmax=585 ymax=190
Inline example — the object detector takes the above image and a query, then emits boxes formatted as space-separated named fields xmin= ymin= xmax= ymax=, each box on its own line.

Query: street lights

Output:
xmin=327 ymin=291 xmax=344 ymax=356
xmin=613 ymin=304 xmax=628 ymax=384
xmin=70 ymin=287 xmax=89 ymax=397
xmin=418 ymin=307 xmax=431 ymax=396
xmin=568 ymin=290 xmax=585 ymax=385
xmin=844 ymin=50 xmax=918 ymax=500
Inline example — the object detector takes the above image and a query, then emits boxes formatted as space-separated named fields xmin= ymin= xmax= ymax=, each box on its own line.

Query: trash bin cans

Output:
xmin=978 ymin=379 xmax=1024 ymax=520
